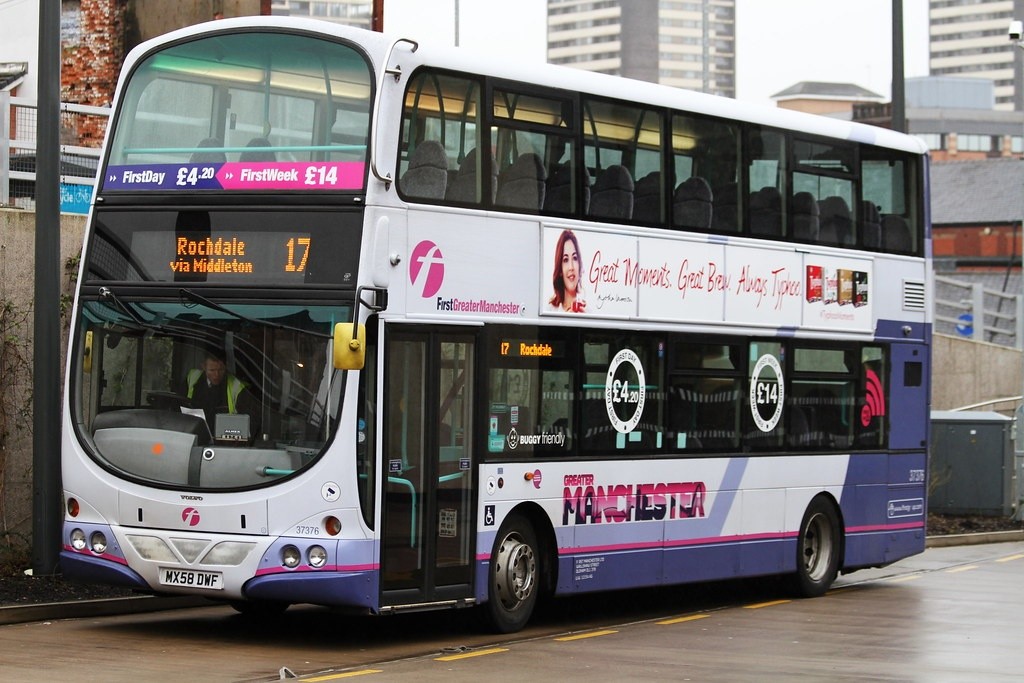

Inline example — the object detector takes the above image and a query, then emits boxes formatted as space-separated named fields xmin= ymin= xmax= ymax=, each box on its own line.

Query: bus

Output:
xmin=57 ymin=13 xmax=931 ymax=631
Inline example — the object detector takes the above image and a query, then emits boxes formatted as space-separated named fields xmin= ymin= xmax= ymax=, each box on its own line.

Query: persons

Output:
xmin=187 ymin=349 xmax=247 ymax=418
xmin=549 ymin=230 xmax=584 ymax=311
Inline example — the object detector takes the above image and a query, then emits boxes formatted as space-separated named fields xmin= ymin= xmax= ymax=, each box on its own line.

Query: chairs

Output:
xmin=398 ymin=141 xmax=913 ymax=256
xmin=239 ymin=138 xmax=276 ymax=162
xmin=544 ymin=388 xmax=845 ymax=455
xmin=497 ymin=406 xmax=534 ymax=452
xmin=190 ymin=137 xmax=228 ymax=162
